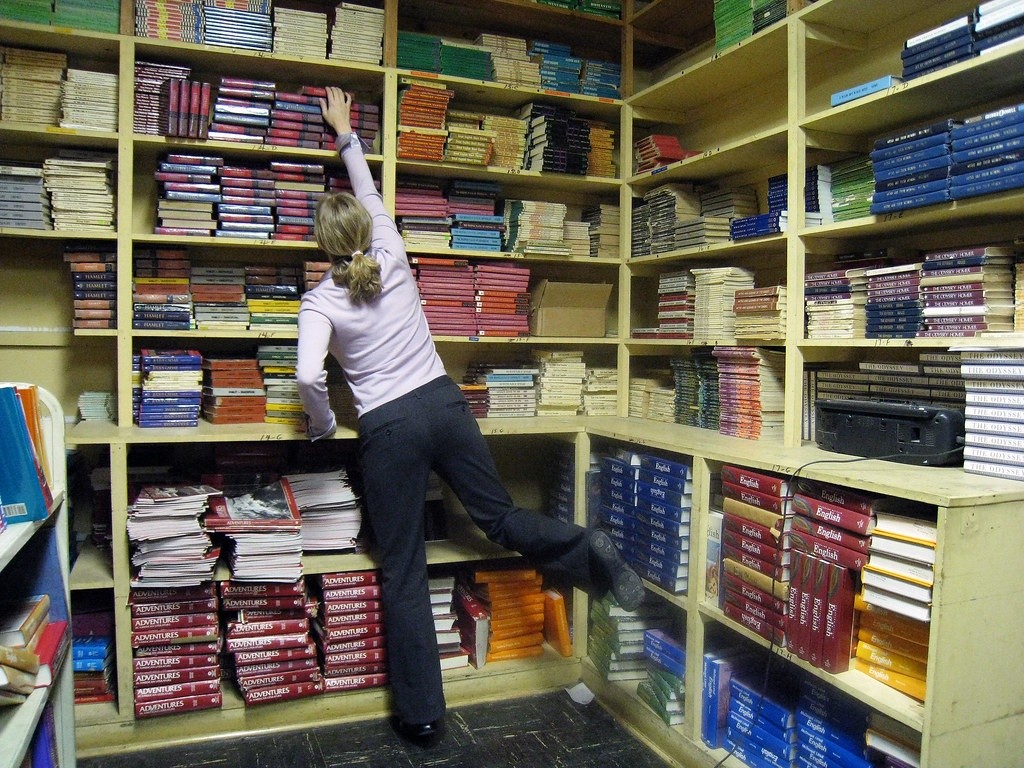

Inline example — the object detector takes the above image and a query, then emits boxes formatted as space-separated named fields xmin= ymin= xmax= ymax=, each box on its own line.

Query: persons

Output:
xmin=295 ymin=83 xmax=651 ymax=749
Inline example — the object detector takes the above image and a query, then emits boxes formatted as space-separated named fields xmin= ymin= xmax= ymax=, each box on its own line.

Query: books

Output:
xmin=585 ymin=1 xmax=1024 ymax=768
xmin=0 ymin=1 xmax=620 ymax=721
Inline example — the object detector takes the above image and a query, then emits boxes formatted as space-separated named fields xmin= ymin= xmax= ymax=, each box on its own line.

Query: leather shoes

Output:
xmin=388 ymin=714 xmax=439 ymax=749
xmin=589 ymin=530 xmax=647 ymax=613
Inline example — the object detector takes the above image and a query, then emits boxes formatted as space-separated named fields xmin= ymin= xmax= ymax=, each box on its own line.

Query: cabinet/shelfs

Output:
xmin=0 ymin=0 xmax=1024 ymax=768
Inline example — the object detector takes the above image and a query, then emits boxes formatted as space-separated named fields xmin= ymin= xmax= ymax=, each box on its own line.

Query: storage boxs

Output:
xmin=527 ymin=277 xmax=613 ymax=337
